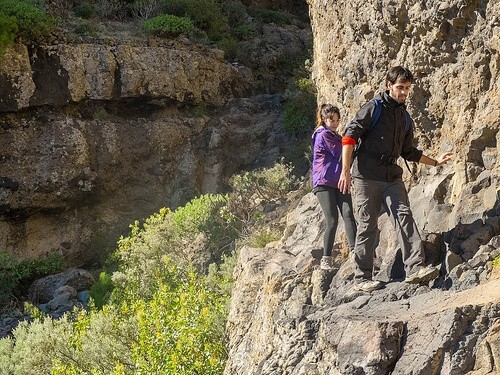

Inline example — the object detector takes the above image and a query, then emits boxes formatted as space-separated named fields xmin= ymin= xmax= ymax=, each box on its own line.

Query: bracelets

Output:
xmin=434 ymin=160 xmax=437 ymax=166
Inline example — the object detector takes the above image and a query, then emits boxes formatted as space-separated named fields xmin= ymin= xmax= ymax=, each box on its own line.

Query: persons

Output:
xmin=312 ymin=104 xmax=357 ymax=270
xmin=338 ymin=66 xmax=453 ymax=292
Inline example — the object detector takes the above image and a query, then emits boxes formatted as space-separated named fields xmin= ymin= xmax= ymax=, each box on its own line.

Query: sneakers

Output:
xmin=404 ymin=267 xmax=438 ymax=284
xmin=354 ymin=279 xmax=382 ymax=292
xmin=320 ymin=256 xmax=338 ymax=271
